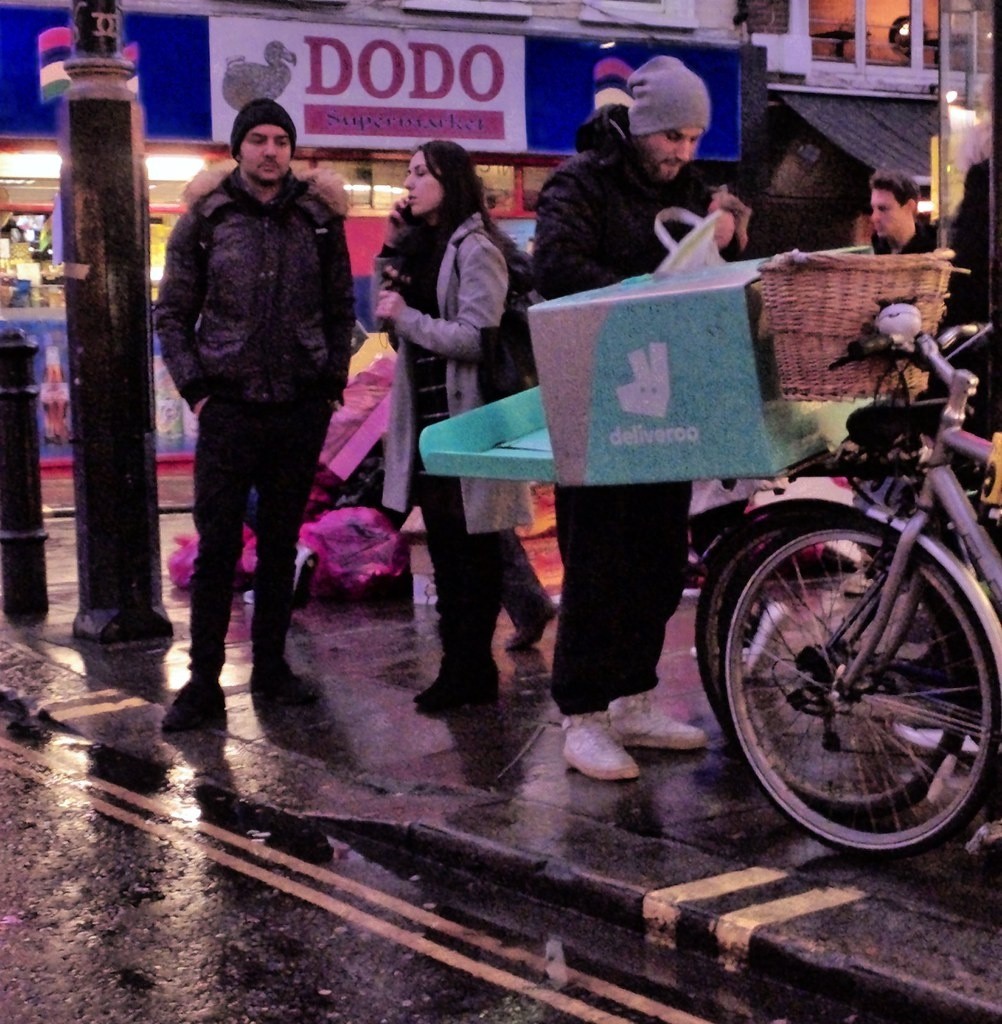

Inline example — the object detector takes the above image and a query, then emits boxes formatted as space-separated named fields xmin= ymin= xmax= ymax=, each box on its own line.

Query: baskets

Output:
xmin=756 ymin=247 xmax=956 ymax=403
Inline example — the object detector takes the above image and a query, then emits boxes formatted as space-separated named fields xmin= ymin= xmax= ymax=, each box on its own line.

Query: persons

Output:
xmin=156 ymin=55 xmax=1001 ymax=852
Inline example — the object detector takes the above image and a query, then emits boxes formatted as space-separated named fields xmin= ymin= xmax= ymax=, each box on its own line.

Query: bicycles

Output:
xmin=694 ymin=298 xmax=1002 ymax=863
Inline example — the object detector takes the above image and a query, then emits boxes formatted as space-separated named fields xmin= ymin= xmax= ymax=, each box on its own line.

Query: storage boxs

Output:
xmin=419 ymin=244 xmax=876 ymax=487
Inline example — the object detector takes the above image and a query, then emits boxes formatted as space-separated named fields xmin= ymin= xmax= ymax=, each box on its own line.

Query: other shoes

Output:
xmin=161 ymin=682 xmax=226 ymax=731
xmin=738 ymin=591 xmax=789 ymax=681
xmin=506 ymin=598 xmax=556 ymax=651
xmin=249 ymin=656 xmax=326 ymax=710
xmin=839 ymin=567 xmax=882 ymax=595
xmin=413 ymin=653 xmax=501 ymax=707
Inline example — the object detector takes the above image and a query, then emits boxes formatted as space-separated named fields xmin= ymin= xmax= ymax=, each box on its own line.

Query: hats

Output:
xmin=627 ymin=56 xmax=710 ymax=136
xmin=230 ymin=98 xmax=296 ymax=161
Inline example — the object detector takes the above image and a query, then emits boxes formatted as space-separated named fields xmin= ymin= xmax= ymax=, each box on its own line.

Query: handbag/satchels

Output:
xmin=656 ymin=207 xmax=727 ymax=274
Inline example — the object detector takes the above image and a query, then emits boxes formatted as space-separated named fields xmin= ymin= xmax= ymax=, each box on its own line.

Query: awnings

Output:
xmin=786 ymin=94 xmax=940 ymax=174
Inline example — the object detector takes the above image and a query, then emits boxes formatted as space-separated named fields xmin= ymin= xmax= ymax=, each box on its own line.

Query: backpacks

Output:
xmin=452 ymin=228 xmax=538 ymax=404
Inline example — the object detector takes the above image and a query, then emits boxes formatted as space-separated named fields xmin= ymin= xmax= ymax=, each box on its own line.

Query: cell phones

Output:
xmin=402 ymin=204 xmax=424 ymax=227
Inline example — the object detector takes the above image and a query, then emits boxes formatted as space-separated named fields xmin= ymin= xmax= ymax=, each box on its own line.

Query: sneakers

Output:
xmin=562 ymin=711 xmax=641 ymax=780
xmin=608 ymin=692 xmax=707 ymax=749
